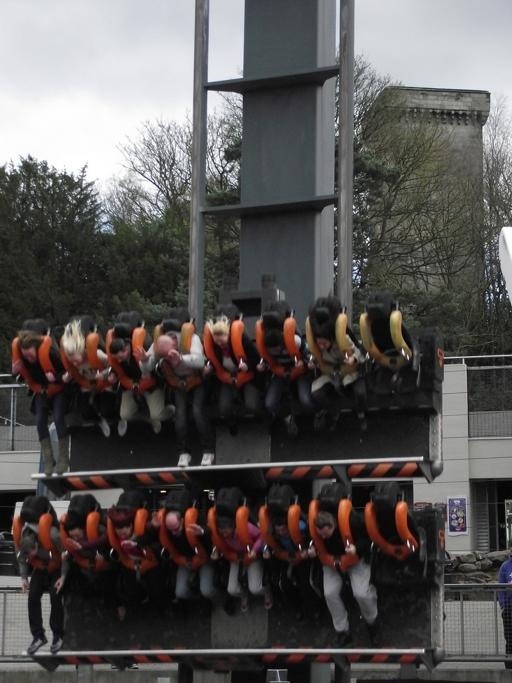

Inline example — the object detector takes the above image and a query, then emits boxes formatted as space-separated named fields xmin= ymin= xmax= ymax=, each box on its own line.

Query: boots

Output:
xmin=39 ymin=436 xmax=56 ymax=475
xmin=55 ymin=434 xmax=70 ymax=476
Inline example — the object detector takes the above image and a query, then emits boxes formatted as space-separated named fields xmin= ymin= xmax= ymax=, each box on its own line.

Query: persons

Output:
xmin=11 ymin=329 xmax=370 ymax=476
xmin=16 ymin=509 xmax=383 ymax=655
xmin=497 ymin=539 xmax=512 ymax=672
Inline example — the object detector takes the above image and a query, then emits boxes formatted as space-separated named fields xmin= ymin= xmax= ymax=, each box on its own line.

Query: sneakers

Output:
xmin=28 ymin=630 xmax=47 ymax=656
xmin=238 ymin=595 xmax=275 ymax=613
xmin=227 ymin=413 xmax=370 ymax=442
xmin=177 ymin=451 xmax=193 ymax=467
xmin=95 ymin=418 xmax=172 ymax=440
xmin=200 ymin=452 xmax=216 ymax=466
xmin=50 ymin=631 xmax=65 ymax=654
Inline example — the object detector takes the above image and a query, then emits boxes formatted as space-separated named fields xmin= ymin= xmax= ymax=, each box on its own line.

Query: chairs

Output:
xmin=15 ymin=292 xmax=428 ymax=616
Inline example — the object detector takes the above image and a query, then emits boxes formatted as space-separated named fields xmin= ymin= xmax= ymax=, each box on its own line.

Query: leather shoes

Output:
xmin=330 ymin=618 xmax=387 ymax=648
